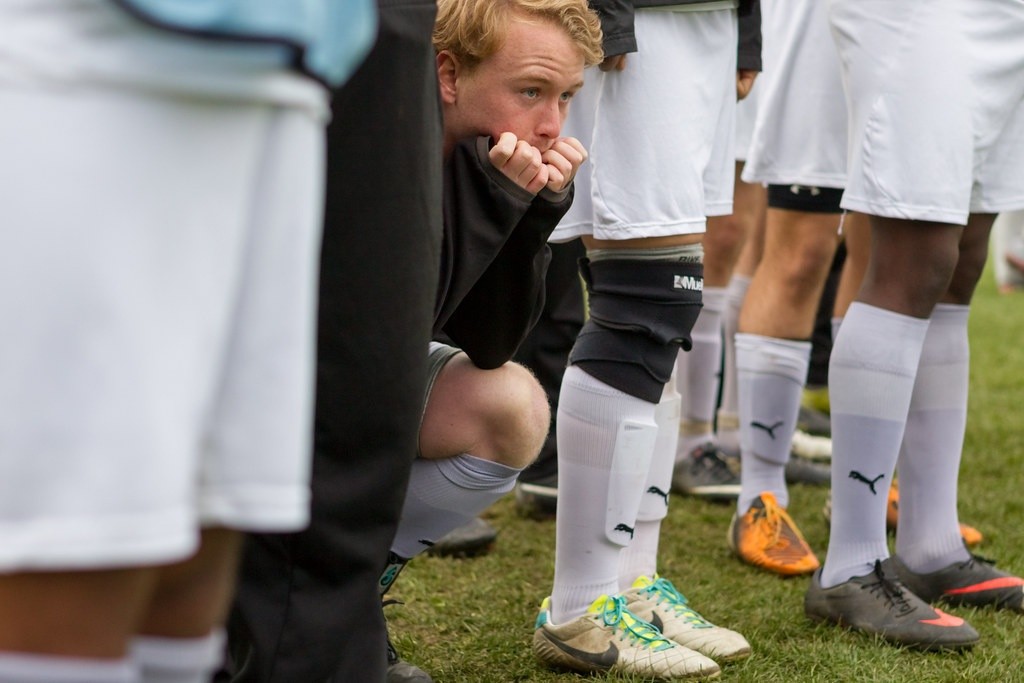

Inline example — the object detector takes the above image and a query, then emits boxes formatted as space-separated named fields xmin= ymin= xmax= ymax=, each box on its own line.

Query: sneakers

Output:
xmin=513 ymin=474 xmax=558 ymax=521
xmin=891 ymin=552 xmax=1024 ymax=614
xmin=530 ymin=597 xmax=721 ymax=683
xmin=802 ymin=559 xmax=980 ymax=650
xmin=674 ymin=442 xmax=741 ymax=500
xmin=784 ymin=430 xmax=831 ymax=485
xmin=725 ymin=493 xmax=822 ymax=576
xmin=822 ymin=476 xmax=980 ymax=550
xmin=622 ymin=574 xmax=753 ymax=662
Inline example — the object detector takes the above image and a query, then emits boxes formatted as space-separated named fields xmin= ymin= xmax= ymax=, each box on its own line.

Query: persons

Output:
xmin=803 ymin=1 xmax=1024 ymax=653
xmin=424 ymin=59 xmax=602 ymax=553
xmin=0 ymin=0 xmax=382 ymax=683
xmin=720 ymin=1 xmax=983 ymax=578
xmin=532 ymin=0 xmax=765 ymax=678
xmin=791 ymin=234 xmax=849 ymax=458
xmin=988 ymin=212 xmax=1024 ymax=295
xmin=670 ymin=70 xmax=832 ymax=501
xmin=218 ymin=0 xmax=438 ymax=683
xmin=381 ymin=0 xmax=608 ymax=683
